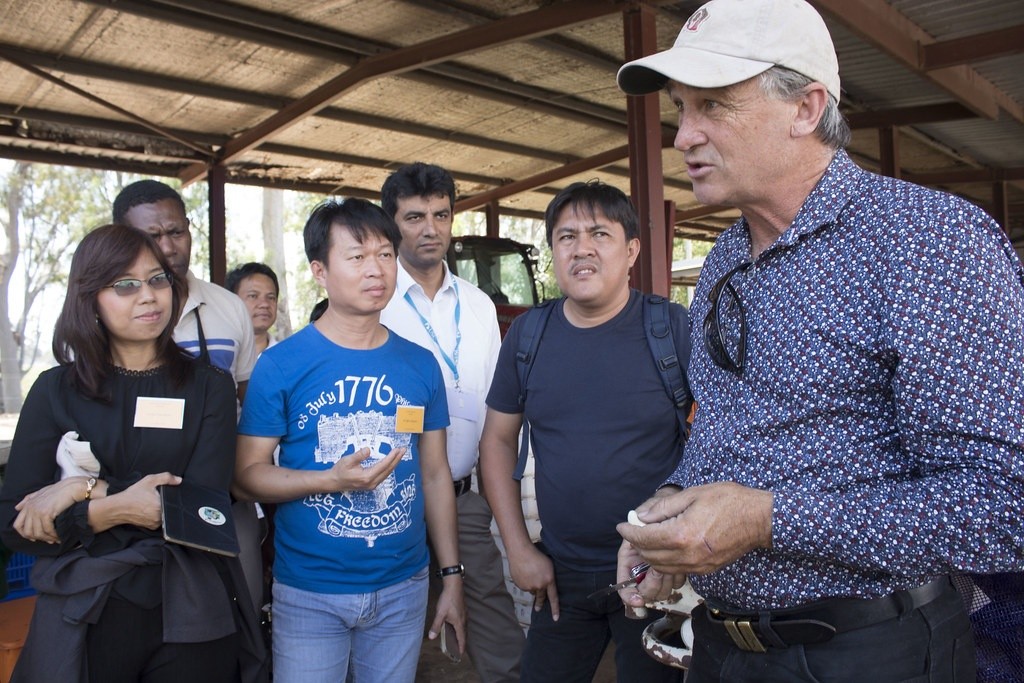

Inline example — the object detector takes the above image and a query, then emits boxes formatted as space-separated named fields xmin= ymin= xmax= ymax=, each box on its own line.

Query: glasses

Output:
xmin=102 ymin=271 xmax=174 ymax=297
xmin=701 ymin=262 xmax=755 ymax=378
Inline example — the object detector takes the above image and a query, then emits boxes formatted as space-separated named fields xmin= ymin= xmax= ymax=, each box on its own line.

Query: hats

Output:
xmin=615 ymin=1 xmax=841 ymax=105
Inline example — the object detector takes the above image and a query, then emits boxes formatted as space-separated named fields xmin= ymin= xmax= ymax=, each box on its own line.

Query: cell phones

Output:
xmin=440 ymin=621 xmax=459 ymax=662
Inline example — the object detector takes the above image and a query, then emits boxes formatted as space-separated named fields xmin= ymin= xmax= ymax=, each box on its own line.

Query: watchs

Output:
xmin=84 ymin=476 xmax=98 ymax=498
xmin=435 ymin=561 xmax=466 ymax=579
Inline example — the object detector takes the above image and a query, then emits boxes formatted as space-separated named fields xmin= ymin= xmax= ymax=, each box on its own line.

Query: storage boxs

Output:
xmin=0 ymin=464 xmax=39 ymax=601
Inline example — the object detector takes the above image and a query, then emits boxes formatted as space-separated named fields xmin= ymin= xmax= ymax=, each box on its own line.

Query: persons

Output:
xmin=616 ymin=0 xmax=1024 ymax=683
xmin=236 ymin=198 xmax=466 ymax=683
xmin=0 ymin=179 xmax=279 ymax=682
xmin=378 ymin=163 xmax=691 ymax=683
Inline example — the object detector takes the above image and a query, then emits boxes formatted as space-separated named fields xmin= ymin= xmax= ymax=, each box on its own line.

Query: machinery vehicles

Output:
xmin=447 ymin=236 xmax=546 ymax=341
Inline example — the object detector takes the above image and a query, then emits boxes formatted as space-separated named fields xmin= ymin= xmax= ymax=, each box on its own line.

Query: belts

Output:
xmin=690 ymin=569 xmax=952 ymax=653
xmin=452 ymin=474 xmax=473 ymax=498
